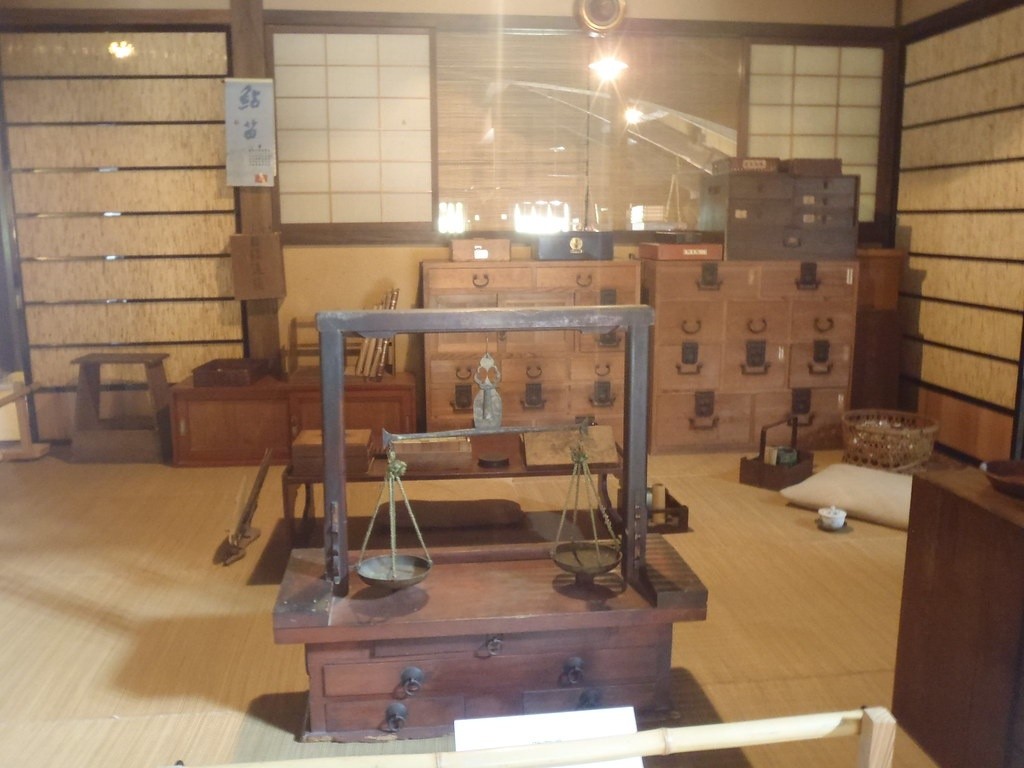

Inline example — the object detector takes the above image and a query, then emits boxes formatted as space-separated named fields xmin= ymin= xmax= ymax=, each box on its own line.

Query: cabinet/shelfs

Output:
xmin=422 ymin=258 xmax=642 ymax=450
xmin=890 ymin=469 xmax=1023 ymax=768
xmin=629 ymin=253 xmax=860 ymax=452
xmin=174 ymin=372 xmax=418 ymax=468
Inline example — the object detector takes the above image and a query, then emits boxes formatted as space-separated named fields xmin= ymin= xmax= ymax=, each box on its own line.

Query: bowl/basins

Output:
xmin=980 ymin=460 xmax=1024 ymax=497
xmin=357 ymin=554 xmax=431 ymax=588
xmin=556 ymin=542 xmax=622 ymax=573
xmin=819 ymin=508 xmax=846 ymax=529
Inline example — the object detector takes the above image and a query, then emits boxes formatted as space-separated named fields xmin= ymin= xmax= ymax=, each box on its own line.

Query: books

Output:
xmin=631 ymin=205 xmax=664 ymax=223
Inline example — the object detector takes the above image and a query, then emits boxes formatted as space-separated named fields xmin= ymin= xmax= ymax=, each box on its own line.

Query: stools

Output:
xmin=69 ymin=353 xmax=170 ymax=462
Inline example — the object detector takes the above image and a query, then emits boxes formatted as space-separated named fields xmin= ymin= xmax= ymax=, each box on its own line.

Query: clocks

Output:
xmin=575 ymin=0 xmax=628 ymax=35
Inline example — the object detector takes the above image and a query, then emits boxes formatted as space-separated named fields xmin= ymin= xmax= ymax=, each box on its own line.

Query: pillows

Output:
xmin=780 ymin=463 xmax=913 ymax=531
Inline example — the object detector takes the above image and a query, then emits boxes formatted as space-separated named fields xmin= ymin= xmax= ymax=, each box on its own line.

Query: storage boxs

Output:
xmin=641 ymin=241 xmax=724 ymax=260
xmin=452 ymin=239 xmax=510 ymax=259
xmin=191 ymin=358 xmax=271 ymax=386
xmin=536 ymin=232 xmax=616 ymax=259
xmin=291 ymin=430 xmax=377 ymax=475
xmin=738 ymin=417 xmax=812 ymax=492
xmin=860 ymin=248 xmax=902 ymax=310
xmin=700 ymin=156 xmax=860 ymax=255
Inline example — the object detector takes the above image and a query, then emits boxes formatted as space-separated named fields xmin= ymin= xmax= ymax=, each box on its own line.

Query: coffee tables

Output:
xmin=280 ymin=427 xmax=623 ymax=539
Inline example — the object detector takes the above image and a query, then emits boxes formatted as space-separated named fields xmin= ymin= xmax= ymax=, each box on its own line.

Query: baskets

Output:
xmin=840 ymin=409 xmax=939 ymax=474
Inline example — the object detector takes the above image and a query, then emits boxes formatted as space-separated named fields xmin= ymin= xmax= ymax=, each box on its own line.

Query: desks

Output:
xmin=272 ymin=531 xmax=709 ymax=739
